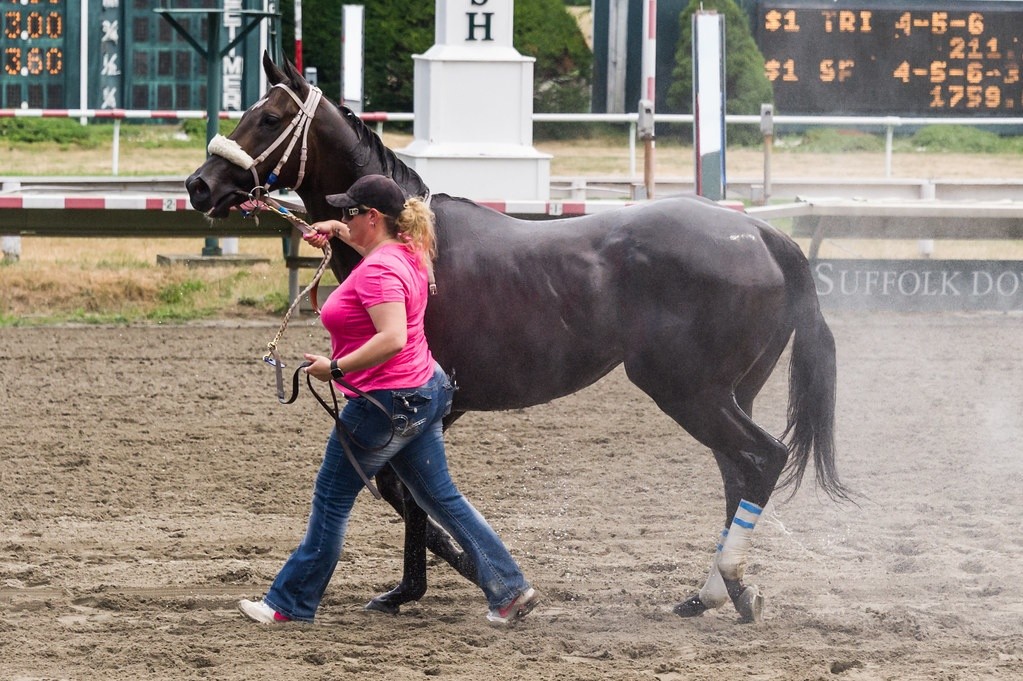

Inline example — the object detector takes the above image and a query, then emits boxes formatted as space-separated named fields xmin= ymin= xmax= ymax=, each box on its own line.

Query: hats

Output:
xmin=325 ymin=174 xmax=407 ymax=218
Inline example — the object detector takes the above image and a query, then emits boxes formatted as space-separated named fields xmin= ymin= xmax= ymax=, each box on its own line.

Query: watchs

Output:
xmin=330 ymin=359 xmax=344 ymax=379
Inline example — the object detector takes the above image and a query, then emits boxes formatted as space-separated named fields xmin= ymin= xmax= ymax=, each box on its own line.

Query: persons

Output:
xmin=237 ymin=174 xmax=540 ymax=624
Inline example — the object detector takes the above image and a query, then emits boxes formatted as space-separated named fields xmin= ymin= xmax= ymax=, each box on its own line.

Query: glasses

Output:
xmin=342 ymin=207 xmax=384 ymax=221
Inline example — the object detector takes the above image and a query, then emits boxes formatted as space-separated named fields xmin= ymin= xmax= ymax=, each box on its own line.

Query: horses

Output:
xmin=184 ymin=48 xmax=837 ymax=624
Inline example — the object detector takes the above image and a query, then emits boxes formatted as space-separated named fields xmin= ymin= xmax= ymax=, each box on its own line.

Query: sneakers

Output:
xmin=236 ymin=598 xmax=292 ymax=623
xmin=485 ymin=586 xmax=540 ymax=624
